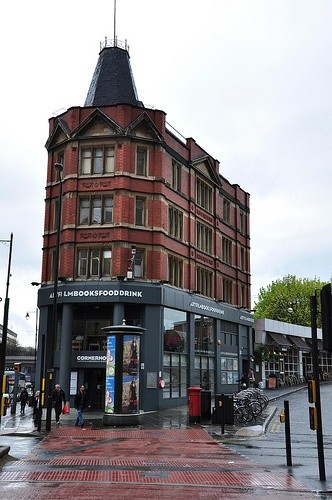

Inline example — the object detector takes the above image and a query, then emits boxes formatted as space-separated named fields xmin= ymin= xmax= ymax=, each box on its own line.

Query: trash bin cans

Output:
xmin=215 ymin=394 xmax=234 ymax=425
xmin=187 ymin=387 xmax=211 ymax=422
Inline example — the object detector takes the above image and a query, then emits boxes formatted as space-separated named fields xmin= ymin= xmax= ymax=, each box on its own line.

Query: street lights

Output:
xmin=25 ymin=308 xmax=38 ymax=376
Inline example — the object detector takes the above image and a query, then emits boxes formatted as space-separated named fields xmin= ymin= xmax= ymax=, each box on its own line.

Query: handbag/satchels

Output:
xmin=62 ymin=403 xmax=69 ymax=414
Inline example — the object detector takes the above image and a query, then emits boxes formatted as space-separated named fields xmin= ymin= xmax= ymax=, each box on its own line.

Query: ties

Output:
xmin=38 ymin=398 xmax=39 ymax=408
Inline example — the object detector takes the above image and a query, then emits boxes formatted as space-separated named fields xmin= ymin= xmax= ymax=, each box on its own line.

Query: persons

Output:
xmin=74 ymin=385 xmax=91 ymax=428
xmin=34 ymin=390 xmax=40 ymax=413
xmin=52 ymin=384 xmax=66 ymax=424
xmin=236 ymin=368 xmax=255 ymax=389
xmin=127 ymin=377 xmax=137 ymax=411
xmin=203 ymin=371 xmax=212 ymax=390
xmin=19 ymin=388 xmax=28 ymax=412
xmin=128 ymin=336 xmax=139 ymax=375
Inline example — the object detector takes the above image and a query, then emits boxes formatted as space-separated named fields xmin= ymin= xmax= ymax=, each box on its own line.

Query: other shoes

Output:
xmin=57 ymin=421 xmax=60 ymax=423
xmin=81 ymin=427 xmax=85 ymax=431
xmin=75 ymin=424 xmax=80 ymax=426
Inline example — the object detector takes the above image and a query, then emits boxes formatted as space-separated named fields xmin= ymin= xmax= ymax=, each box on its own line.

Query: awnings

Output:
xmin=269 ymin=333 xmax=328 ymax=351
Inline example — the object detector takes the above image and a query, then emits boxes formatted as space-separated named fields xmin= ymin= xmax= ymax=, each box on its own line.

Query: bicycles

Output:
xmin=213 ymin=387 xmax=270 ymax=425
xmin=275 ymin=374 xmax=306 ymax=388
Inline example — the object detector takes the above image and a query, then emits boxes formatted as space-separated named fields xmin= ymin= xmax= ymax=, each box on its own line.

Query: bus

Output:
xmin=4 ymin=370 xmax=26 ymax=403
